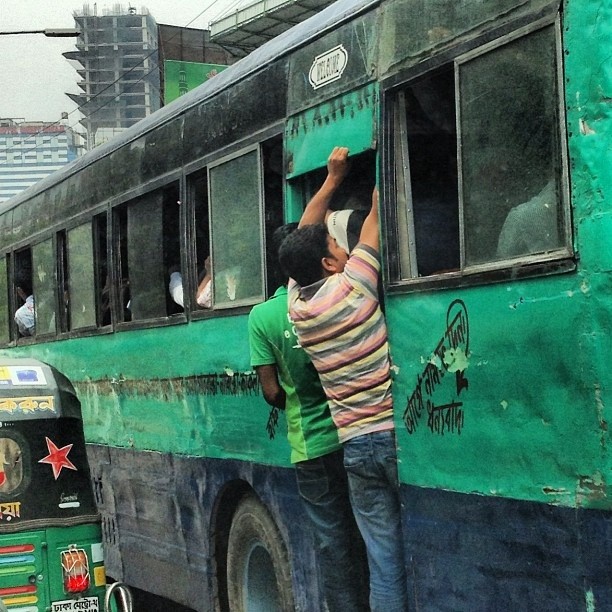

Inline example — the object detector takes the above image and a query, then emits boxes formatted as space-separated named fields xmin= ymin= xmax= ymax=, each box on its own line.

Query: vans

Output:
xmin=0 ymin=357 xmax=133 ymax=612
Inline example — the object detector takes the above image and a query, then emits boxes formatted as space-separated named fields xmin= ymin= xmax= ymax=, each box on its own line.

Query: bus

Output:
xmin=0 ymin=0 xmax=612 ymax=612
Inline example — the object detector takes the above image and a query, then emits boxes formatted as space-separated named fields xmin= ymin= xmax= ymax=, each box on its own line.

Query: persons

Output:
xmin=324 ymin=209 xmax=369 ymax=255
xmin=13 ymin=261 xmax=35 ymax=335
xmin=195 ymin=241 xmax=246 ymax=308
xmin=278 ymin=147 xmax=408 ymax=612
xmin=248 ymin=223 xmax=369 ymax=612
xmin=495 ymin=137 xmax=558 ymax=259
xmin=163 ymin=227 xmax=184 ymax=308
